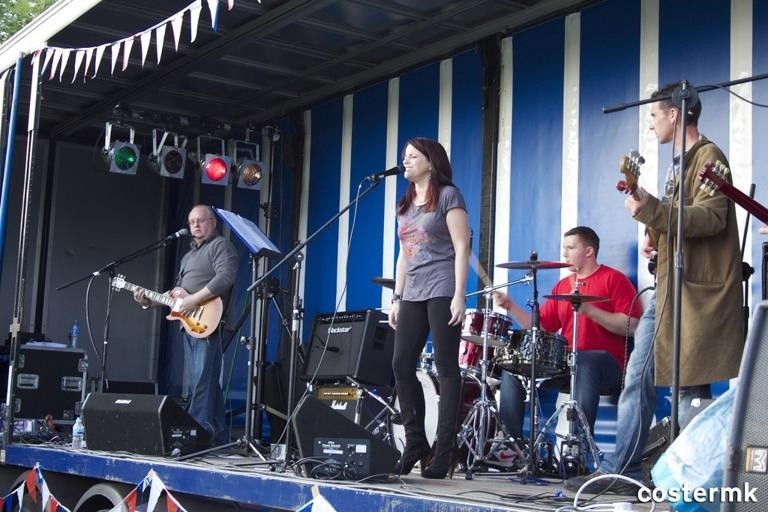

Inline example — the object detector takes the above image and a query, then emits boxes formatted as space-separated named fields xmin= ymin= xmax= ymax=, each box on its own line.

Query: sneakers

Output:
xmin=490 ymin=441 xmax=523 ymax=471
xmin=560 ymin=456 xmax=589 ymax=481
xmin=565 ymin=469 xmax=651 ymax=496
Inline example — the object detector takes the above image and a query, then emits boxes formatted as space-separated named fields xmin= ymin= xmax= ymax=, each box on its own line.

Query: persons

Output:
xmin=760 ymin=223 xmax=768 ymax=235
xmin=490 ymin=226 xmax=644 ymax=476
xmin=563 ymin=80 xmax=746 ymax=496
xmin=389 ymin=136 xmax=470 ymax=482
xmin=132 ymin=206 xmax=240 ymax=453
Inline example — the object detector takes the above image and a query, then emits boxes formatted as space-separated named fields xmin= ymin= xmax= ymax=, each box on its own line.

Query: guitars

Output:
xmin=617 ymin=148 xmax=658 ymax=278
xmin=697 ymin=159 xmax=764 ymax=224
xmin=111 ymin=274 xmax=223 ymax=338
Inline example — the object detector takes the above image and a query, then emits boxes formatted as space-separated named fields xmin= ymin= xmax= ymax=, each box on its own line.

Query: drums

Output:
xmin=493 ymin=330 xmax=568 ymax=378
xmin=461 ymin=308 xmax=512 ymax=346
xmin=459 ymin=339 xmax=501 ymax=386
xmin=387 ymin=366 xmax=499 ymax=472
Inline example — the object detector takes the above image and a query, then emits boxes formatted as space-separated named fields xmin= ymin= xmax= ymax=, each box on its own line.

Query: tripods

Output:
xmin=458 ymin=313 xmax=604 ymax=483
xmin=175 ymin=284 xmax=279 ymax=478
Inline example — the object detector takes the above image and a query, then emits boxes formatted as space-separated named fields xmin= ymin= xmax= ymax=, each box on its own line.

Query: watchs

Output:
xmin=388 ymin=293 xmax=403 ymax=303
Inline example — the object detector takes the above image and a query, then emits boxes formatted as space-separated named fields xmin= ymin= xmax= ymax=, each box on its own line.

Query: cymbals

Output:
xmin=543 ymin=294 xmax=609 ymax=302
xmin=496 ymin=261 xmax=576 ymax=268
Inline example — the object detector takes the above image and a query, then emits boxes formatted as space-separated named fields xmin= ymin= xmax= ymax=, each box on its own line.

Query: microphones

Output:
xmin=367 ymin=163 xmax=405 ymax=181
xmin=163 ymin=228 xmax=188 ymax=242
xmin=317 ymin=342 xmax=340 ymax=355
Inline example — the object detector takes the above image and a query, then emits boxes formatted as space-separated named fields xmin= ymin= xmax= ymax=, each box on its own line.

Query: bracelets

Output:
xmin=142 ymin=300 xmax=152 ymax=310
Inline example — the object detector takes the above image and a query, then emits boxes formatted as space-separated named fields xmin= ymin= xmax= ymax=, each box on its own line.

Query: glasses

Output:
xmin=187 ymin=218 xmax=214 ymax=225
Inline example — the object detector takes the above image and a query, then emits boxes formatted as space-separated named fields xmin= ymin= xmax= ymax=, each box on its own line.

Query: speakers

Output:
xmin=308 ymin=382 xmax=393 ymax=446
xmin=304 ymin=308 xmax=396 ymax=385
xmin=313 ymin=437 xmax=402 ymax=479
xmin=83 ymin=392 xmax=209 ymax=460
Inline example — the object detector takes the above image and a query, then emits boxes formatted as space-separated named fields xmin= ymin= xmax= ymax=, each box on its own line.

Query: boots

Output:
xmin=392 ymin=381 xmax=431 ymax=475
xmin=419 ymin=379 xmax=462 ymax=479
